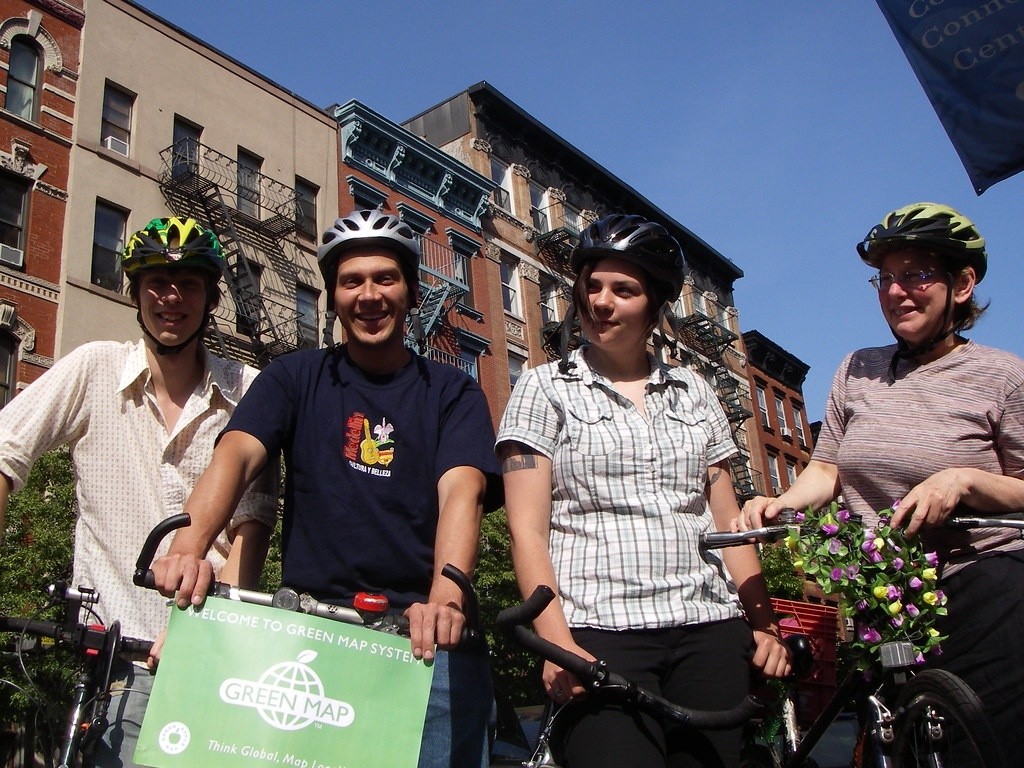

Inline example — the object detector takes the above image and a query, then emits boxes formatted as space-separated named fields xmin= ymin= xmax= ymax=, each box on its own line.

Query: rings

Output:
xmin=554 ymin=688 xmax=563 ymax=696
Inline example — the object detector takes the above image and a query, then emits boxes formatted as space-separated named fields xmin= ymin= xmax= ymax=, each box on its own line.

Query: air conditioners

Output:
xmin=0 ymin=243 xmax=24 ymax=270
xmin=102 ymin=135 xmax=130 ymax=158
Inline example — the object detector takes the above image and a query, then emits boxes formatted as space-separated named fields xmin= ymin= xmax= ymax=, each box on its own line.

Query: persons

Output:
xmin=731 ymin=203 xmax=1024 ymax=767
xmin=493 ymin=215 xmax=792 ymax=768
xmin=0 ymin=218 xmax=281 ymax=766
xmin=151 ymin=211 xmax=503 ymax=767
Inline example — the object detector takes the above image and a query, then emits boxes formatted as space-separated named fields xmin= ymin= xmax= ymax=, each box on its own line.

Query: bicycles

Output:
xmin=700 ymin=515 xmax=1024 ymax=762
xmin=497 ymin=583 xmax=764 ymax=768
xmin=135 ymin=512 xmax=482 ymax=661
xmin=1 ymin=572 xmax=156 ymax=768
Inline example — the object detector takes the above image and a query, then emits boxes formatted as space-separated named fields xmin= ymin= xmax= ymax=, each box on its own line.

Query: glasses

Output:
xmin=868 ymin=267 xmax=958 ymax=291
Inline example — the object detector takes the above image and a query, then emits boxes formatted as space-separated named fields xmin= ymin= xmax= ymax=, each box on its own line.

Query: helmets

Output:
xmin=317 ymin=210 xmax=421 ymax=284
xmin=569 ymin=214 xmax=685 ymax=304
xmin=120 ymin=216 xmax=224 ymax=283
xmin=856 ymin=202 xmax=988 ymax=286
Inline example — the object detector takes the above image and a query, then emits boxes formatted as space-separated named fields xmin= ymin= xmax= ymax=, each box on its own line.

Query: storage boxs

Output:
xmin=751 ymin=597 xmax=839 ymax=727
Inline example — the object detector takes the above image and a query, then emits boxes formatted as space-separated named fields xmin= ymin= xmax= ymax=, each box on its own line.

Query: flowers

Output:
xmin=791 ymin=495 xmax=951 ymax=665
